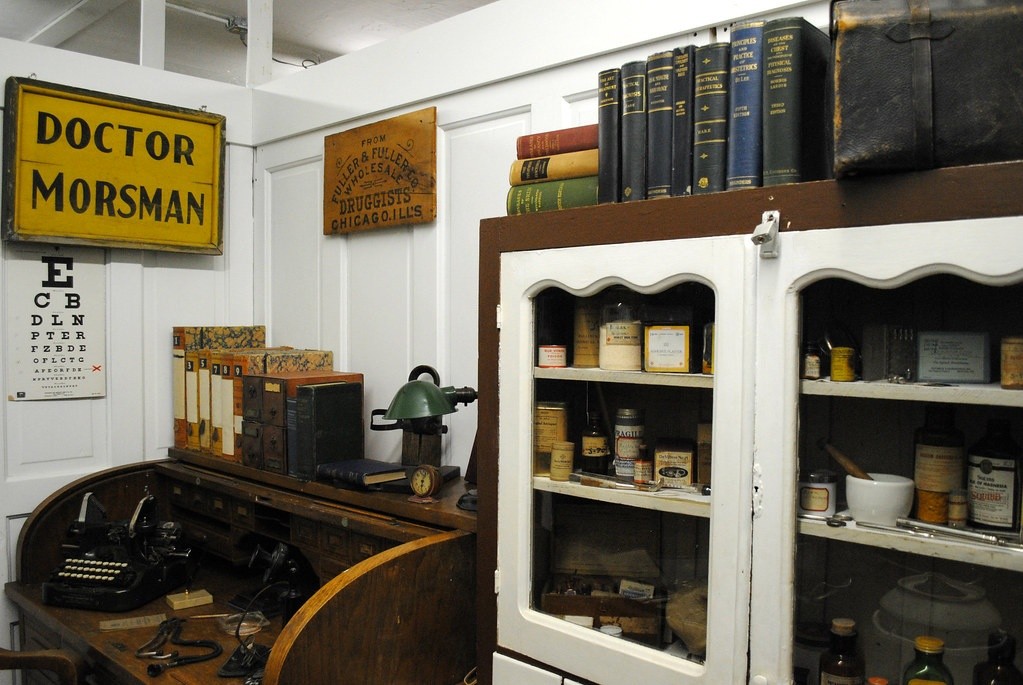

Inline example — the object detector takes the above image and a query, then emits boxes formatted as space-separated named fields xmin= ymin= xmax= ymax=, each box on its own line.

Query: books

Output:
xmin=173 ymin=326 xmax=364 ymax=483
xmin=646 ymin=51 xmax=673 ymax=199
xmin=692 ymin=42 xmax=730 ymax=195
xmin=509 ymin=149 xmax=598 ymax=186
xmin=763 ymin=17 xmax=829 ymax=186
xmin=598 ymin=68 xmax=621 ymax=204
xmin=516 ymin=124 xmax=599 ymax=160
xmin=726 ymin=20 xmax=766 ymax=191
xmin=621 ymin=61 xmax=646 ymax=203
xmin=506 ymin=176 xmax=598 ymax=216
xmin=317 ymin=459 xmax=407 ymax=486
xmin=670 ymin=45 xmax=696 ymax=197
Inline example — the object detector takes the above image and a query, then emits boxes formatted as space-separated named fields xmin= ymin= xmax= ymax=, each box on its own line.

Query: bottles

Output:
xmin=793 ymin=401 xmax=1023 ymax=685
xmin=531 ymin=286 xmax=715 ymax=488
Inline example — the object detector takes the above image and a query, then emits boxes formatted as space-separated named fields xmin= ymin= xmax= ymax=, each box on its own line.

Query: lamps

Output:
xmin=369 ymin=364 xmax=478 ymax=496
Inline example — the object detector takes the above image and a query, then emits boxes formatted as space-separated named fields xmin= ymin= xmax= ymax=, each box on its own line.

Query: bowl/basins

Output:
xmin=844 ymin=472 xmax=914 ymax=527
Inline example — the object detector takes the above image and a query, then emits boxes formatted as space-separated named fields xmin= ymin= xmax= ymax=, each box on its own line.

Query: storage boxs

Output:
xmin=653 ymin=444 xmax=693 ymax=490
xmin=696 ymin=422 xmax=712 ymax=485
xmin=539 ymin=572 xmax=666 ymax=652
xmin=644 ymin=324 xmax=692 ymax=372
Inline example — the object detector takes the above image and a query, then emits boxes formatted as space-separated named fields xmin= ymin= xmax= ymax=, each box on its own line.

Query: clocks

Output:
xmin=407 ymin=464 xmax=441 ymax=504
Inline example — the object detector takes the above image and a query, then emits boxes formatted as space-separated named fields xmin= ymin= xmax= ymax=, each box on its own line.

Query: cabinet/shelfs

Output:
xmin=4 ymin=446 xmax=477 ymax=684
xmin=479 ymin=161 xmax=1023 ymax=685
